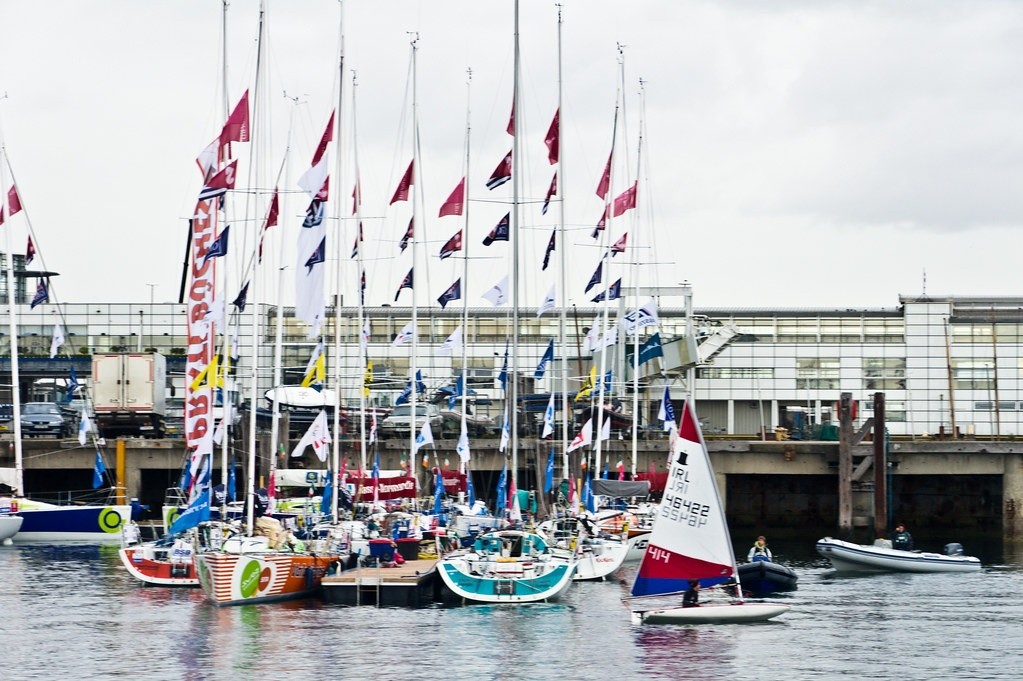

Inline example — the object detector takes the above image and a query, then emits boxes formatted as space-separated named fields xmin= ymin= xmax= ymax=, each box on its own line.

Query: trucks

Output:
xmin=89 ymin=351 xmax=169 ymax=438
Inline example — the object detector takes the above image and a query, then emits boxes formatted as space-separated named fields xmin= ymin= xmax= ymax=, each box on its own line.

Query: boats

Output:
xmin=729 ymin=560 xmax=800 ymax=592
xmin=813 ymin=536 xmax=984 ymax=574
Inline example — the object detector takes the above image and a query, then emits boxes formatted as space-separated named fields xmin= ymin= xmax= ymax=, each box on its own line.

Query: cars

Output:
xmin=18 ymin=401 xmax=69 ymax=438
xmin=380 ymin=402 xmax=445 ymax=441
xmin=1 ymin=404 xmax=14 ymax=420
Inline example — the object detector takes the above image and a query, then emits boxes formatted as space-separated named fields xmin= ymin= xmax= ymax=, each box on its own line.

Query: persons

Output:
xmin=683 ymin=578 xmax=702 ymax=607
xmin=889 ymin=524 xmax=913 ymax=551
xmin=748 ymin=536 xmax=772 ymax=562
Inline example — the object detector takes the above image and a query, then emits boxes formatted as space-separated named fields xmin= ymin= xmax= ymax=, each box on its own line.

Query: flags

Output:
xmin=0 ymin=184 xmax=106 ymax=489
xmin=169 ymin=90 xmax=678 ymax=535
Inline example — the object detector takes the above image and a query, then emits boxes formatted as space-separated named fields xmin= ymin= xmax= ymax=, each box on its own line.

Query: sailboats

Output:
xmin=100 ymin=0 xmax=670 ymax=606
xmin=616 ymin=390 xmax=790 ymax=624
xmin=0 ymin=143 xmax=149 ymax=551
